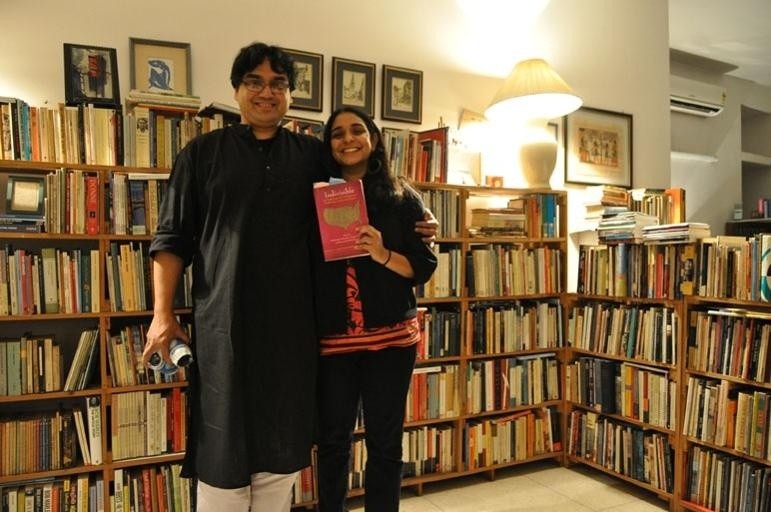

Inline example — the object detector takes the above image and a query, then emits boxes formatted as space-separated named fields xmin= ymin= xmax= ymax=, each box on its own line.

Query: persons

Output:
xmin=313 ymin=103 xmax=439 ymax=512
xmin=142 ymin=41 xmax=439 ymax=512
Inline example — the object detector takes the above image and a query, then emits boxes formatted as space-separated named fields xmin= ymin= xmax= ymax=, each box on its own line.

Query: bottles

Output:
xmin=145 ymin=350 xmax=177 ymax=376
xmin=733 ymin=202 xmax=743 ymax=220
xmin=751 ymin=194 xmax=771 ymax=220
xmin=167 ymin=338 xmax=193 ymax=370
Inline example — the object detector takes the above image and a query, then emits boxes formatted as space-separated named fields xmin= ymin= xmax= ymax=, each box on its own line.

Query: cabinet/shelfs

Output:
xmin=568 ymin=291 xmax=770 ymax=512
xmin=0 ymin=160 xmax=569 ymax=512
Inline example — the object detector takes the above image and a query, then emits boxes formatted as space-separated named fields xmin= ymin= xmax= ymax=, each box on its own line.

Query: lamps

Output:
xmin=484 ymin=59 xmax=583 ymax=189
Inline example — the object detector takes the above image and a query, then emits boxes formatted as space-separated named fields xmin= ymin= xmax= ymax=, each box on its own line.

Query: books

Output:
xmin=1 ymin=93 xmax=243 ymax=169
xmin=420 ymin=189 xmax=460 ymax=238
xmin=414 ymin=243 xmax=461 ymax=298
xmin=401 ymin=424 xmax=457 ymax=478
xmin=291 ymin=396 xmax=370 ymax=505
xmin=279 ymin=115 xmax=326 ymax=143
xmin=313 ymin=178 xmax=373 ymax=261
xmin=383 ymin=128 xmax=447 ymax=184
xmin=404 ymin=363 xmax=462 ymax=422
xmin=466 ymin=243 xmax=561 ymax=296
xmin=465 ymin=405 xmax=561 ymax=470
xmin=466 ymin=354 xmax=561 ymax=413
xmin=467 ymin=194 xmax=556 ymax=238
xmin=2 ymin=166 xmax=194 ymax=512
xmin=0 ymin=90 xmax=243 ymax=512
xmin=567 ymin=185 xmax=770 ymax=512
xmin=416 ymin=306 xmax=461 ymax=359
xmin=467 ymin=299 xmax=565 ymax=355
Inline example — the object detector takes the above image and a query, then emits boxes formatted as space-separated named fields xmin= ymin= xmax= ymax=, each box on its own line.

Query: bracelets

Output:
xmin=384 ymin=250 xmax=393 ymax=265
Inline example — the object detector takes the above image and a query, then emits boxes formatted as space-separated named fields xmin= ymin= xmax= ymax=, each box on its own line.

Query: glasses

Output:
xmin=240 ymin=79 xmax=292 ymax=94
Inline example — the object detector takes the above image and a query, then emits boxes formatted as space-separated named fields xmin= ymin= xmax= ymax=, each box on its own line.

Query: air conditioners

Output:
xmin=670 ymin=74 xmax=725 ymax=117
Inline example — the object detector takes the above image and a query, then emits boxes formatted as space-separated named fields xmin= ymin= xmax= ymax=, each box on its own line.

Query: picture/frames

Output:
xmin=63 ymin=42 xmax=121 ymax=107
xmin=382 ymin=64 xmax=423 ymax=124
xmin=332 ymin=56 xmax=376 ymax=119
xmin=564 ymin=105 xmax=632 ymax=190
xmin=129 ymin=37 xmax=193 ymax=97
xmin=279 ymin=48 xmax=324 ymax=112
